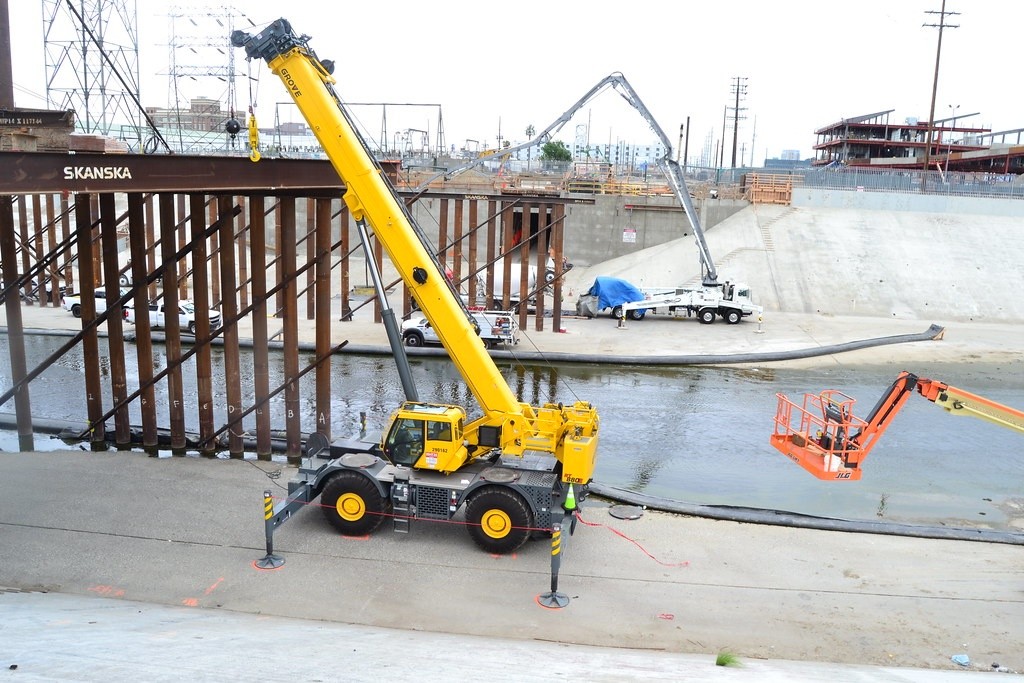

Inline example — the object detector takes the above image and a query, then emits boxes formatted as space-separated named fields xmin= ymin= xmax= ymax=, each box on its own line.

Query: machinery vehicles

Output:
xmin=769 ymin=370 xmax=1024 ymax=485
xmin=414 ymin=67 xmax=769 ymax=326
xmin=229 ymin=16 xmax=599 ymax=609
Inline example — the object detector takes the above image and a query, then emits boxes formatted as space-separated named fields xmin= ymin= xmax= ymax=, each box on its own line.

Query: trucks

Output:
xmin=400 ymin=307 xmax=519 ymax=350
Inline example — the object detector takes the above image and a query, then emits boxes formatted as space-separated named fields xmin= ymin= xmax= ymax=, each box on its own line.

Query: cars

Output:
xmin=119 ymin=256 xmax=190 ymax=287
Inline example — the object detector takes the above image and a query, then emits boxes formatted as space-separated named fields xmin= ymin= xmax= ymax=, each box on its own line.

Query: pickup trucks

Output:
xmin=61 ymin=285 xmax=139 ymax=317
xmin=0 ymin=275 xmax=68 ymax=304
xmin=125 ymin=300 xmax=223 ymax=336
